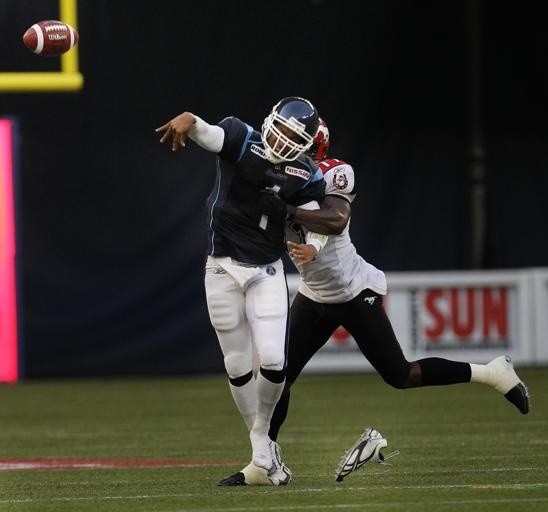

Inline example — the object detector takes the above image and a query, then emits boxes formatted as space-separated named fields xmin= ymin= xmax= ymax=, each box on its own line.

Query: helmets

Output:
xmin=261 ymin=96 xmax=331 ymax=165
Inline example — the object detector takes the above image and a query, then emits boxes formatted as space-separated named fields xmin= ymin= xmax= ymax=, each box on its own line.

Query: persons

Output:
xmin=219 ymin=117 xmax=530 ymax=486
xmin=156 ymin=96 xmax=329 ymax=485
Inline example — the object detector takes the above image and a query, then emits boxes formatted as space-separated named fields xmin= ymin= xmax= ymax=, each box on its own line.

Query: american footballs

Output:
xmin=24 ymin=20 xmax=80 ymax=55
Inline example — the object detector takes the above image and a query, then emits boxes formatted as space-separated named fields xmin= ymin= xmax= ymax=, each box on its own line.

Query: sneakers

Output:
xmin=335 ymin=427 xmax=389 ymax=482
xmin=218 ymin=442 xmax=292 ymax=487
xmin=489 ymin=356 xmax=530 ymax=415
